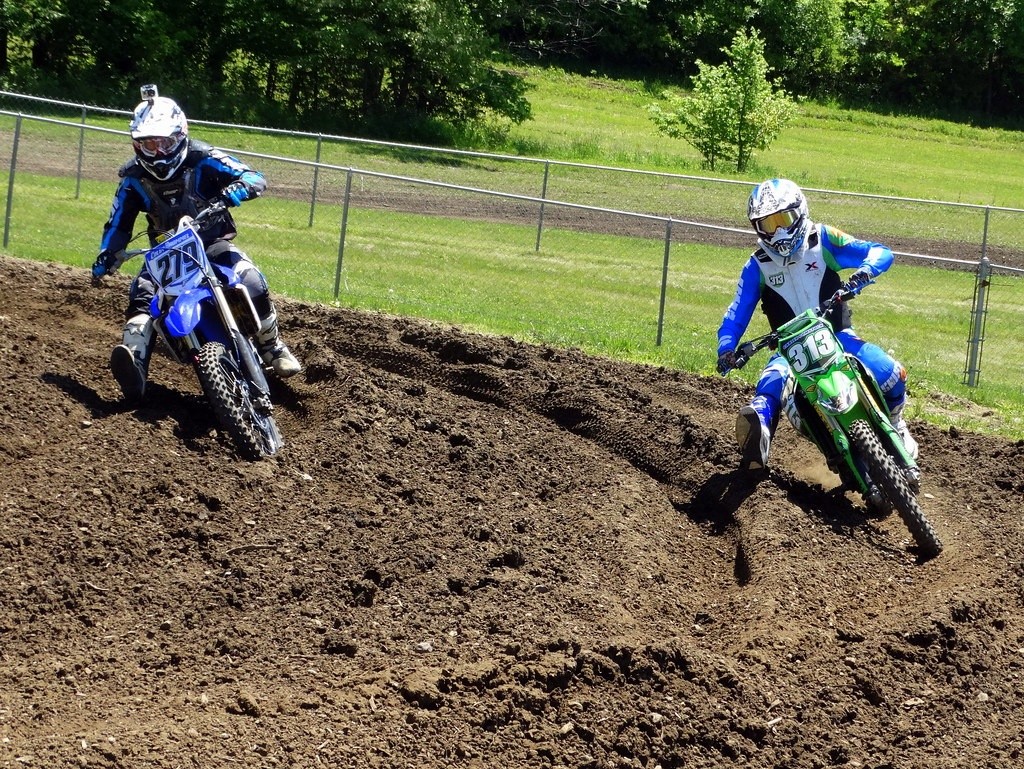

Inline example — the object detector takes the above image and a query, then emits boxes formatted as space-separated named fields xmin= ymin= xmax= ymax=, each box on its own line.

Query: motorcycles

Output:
xmin=716 ymin=273 xmax=944 ymax=556
xmin=91 ymin=187 xmax=285 ymax=462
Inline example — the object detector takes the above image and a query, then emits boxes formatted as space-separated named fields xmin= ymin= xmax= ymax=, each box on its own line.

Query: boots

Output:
xmin=111 ymin=313 xmax=157 ymax=399
xmin=253 ymin=302 xmax=302 ymax=378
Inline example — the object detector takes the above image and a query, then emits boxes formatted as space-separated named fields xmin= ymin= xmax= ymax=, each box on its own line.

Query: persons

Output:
xmin=93 ymin=96 xmax=301 ymax=399
xmin=718 ymin=178 xmax=918 ymax=502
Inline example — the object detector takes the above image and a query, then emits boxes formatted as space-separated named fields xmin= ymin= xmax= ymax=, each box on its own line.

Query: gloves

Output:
xmin=716 ymin=350 xmax=735 ymax=372
xmin=849 ymin=265 xmax=870 ymax=295
xmin=221 ymin=183 xmax=248 ymax=207
xmin=92 ymin=251 xmax=117 ymax=276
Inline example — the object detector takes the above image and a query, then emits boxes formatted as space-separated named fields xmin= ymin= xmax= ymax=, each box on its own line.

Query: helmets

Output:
xmin=129 ymin=97 xmax=188 ymax=181
xmin=747 ymin=179 xmax=810 ymax=257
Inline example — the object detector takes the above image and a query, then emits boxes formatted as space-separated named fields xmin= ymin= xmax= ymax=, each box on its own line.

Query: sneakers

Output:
xmin=889 ymin=410 xmax=918 ymax=460
xmin=735 ymin=406 xmax=770 ymax=469
